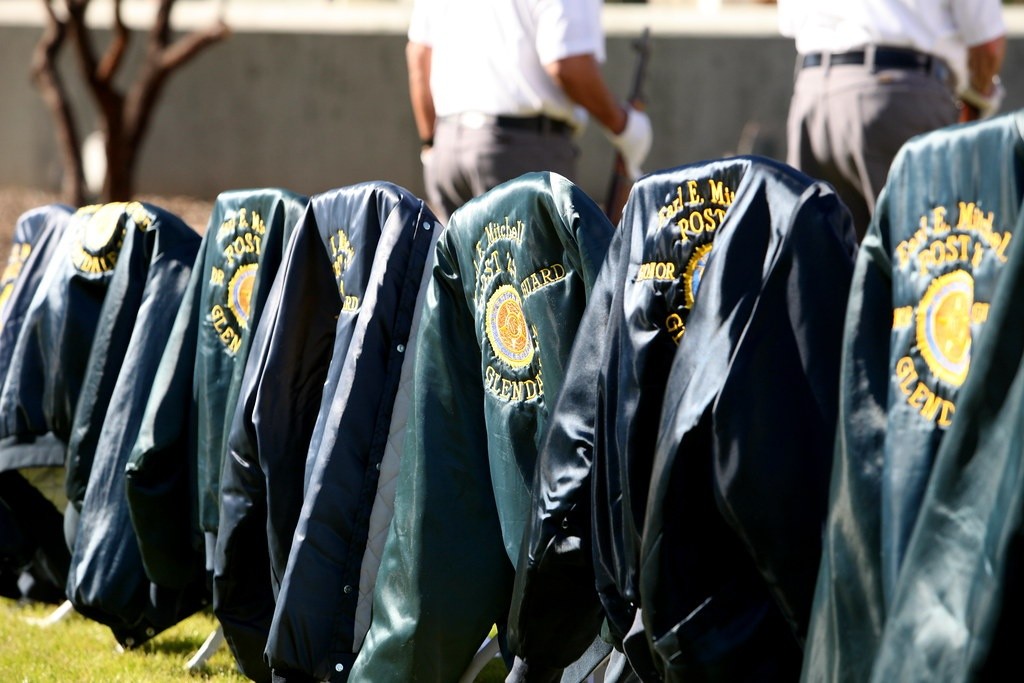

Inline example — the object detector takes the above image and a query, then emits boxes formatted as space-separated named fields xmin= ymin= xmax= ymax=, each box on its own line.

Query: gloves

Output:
xmin=605 ymin=105 xmax=652 ymax=183
xmin=957 ymin=68 xmax=1007 ymax=121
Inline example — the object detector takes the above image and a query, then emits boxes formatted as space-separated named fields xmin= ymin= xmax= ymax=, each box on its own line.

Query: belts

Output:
xmin=801 ymin=45 xmax=953 ymax=85
xmin=436 ymin=112 xmax=578 ymax=138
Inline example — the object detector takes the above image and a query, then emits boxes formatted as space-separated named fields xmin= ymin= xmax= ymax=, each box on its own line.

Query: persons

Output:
xmin=404 ymin=2 xmax=654 ymax=228
xmin=773 ymin=0 xmax=1005 ymax=217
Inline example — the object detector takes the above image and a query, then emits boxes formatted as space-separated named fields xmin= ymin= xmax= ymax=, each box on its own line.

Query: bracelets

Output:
xmin=419 ymin=136 xmax=434 ymax=147
